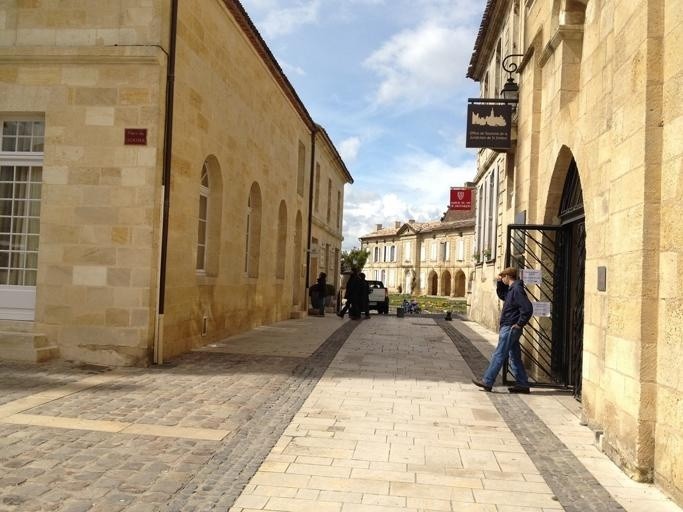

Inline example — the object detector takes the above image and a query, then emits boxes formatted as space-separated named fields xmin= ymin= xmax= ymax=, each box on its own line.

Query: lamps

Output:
xmin=499 ymin=51 xmax=528 ymax=110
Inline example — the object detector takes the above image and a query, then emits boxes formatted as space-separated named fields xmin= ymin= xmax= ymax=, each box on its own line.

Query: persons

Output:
xmin=316 ymin=271 xmax=328 ymax=316
xmin=358 ymin=273 xmax=371 ymax=320
xmin=471 ymin=267 xmax=533 ymax=395
xmin=335 ymin=267 xmax=361 ymax=320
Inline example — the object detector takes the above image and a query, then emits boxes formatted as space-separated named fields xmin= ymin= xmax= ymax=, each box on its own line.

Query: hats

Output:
xmin=498 ymin=267 xmax=518 ymax=277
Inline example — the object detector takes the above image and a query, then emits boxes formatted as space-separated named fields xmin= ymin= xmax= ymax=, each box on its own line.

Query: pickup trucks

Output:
xmin=365 ymin=280 xmax=389 ymax=315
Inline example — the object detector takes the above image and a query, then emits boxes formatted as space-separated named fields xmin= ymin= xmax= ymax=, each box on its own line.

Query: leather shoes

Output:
xmin=472 ymin=376 xmax=493 ymax=392
xmin=508 ymin=385 xmax=530 ymax=394
